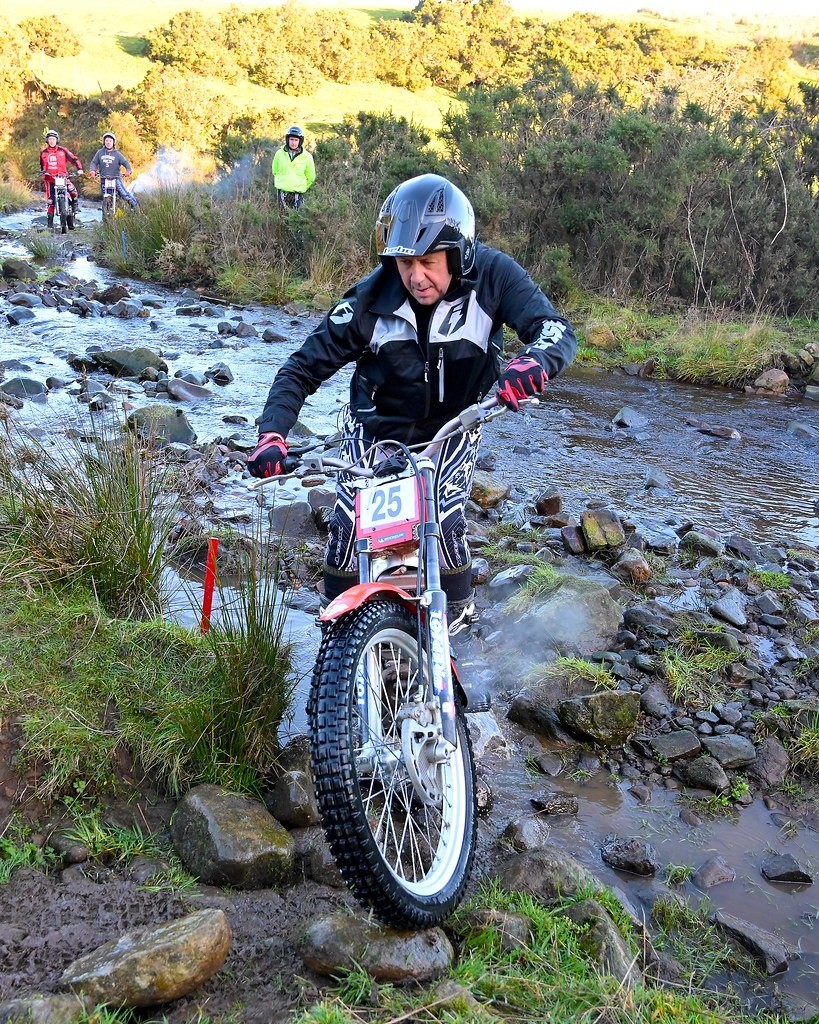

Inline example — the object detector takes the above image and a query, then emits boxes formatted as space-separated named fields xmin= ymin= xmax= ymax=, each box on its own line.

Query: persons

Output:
xmin=272 ymin=126 xmax=316 ymax=209
xmin=39 ymin=130 xmax=84 ymax=228
xmin=247 ymin=172 xmax=577 ymax=712
xmin=89 ymin=132 xmax=141 ymax=216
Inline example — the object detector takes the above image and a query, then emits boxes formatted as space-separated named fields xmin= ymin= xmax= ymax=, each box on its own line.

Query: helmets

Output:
xmin=285 ymin=127 xmax=304 ymax=148
xmin=45 ymin=130 xmax=60 ymax=143
xmin=102 ymin=132 xmax=117 ymax=145
xmin=375 ymin=173 xmax=476 ymax=280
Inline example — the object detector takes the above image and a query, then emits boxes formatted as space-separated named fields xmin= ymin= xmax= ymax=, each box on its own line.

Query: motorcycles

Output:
xmin=236 ymin=394 xmax=540 ymax=930
xmin=38 ymin=170 xmax=84 ymax=234
xmin=92 ymin=172 xmax=131 ymax=226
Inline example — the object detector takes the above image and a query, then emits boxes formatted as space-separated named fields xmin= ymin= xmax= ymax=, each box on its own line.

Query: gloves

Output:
xmin=125 ymin=170 xmax=131 ymax=177
xmin=77 ymin=169 xmax=84 ymax=177
xmin=90 ymin=171 xmax=99 ymax=178
xmin=247 ymin=431 xmax=288 ymax=485
xmin=43 ymin=171 xmax=51 ymax=176
xmin=496 ymin=356 xmax=548 ymax=412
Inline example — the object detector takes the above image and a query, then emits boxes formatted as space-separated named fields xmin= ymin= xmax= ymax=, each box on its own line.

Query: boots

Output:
xmin=447 ymin=589 xmax=491 ymax=714
xmin=71 ymin=195 xmax=82 ymax=212
xmin=47 ymin=213 xmax=54 ymax=230
